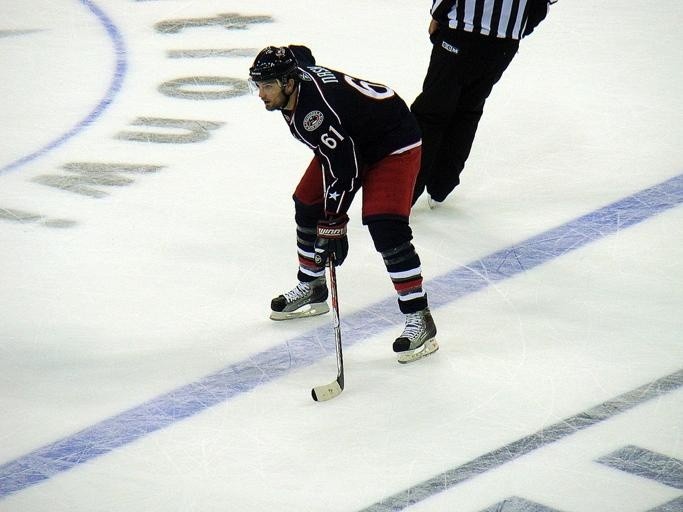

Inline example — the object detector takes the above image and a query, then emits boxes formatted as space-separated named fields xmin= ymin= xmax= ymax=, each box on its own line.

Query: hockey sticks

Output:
xmin=311 ymin=164 xmax=345 ymax=401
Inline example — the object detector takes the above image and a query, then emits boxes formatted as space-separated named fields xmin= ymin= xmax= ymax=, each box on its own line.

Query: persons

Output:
xmin=410 ymin=0 xmax=559 ymax=208
xmin=249 ymin=45 xmax=437 ymax=352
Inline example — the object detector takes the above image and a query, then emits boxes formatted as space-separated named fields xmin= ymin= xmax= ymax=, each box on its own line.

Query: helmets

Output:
xmin=249 ymin=46 xmax=298 ymax=81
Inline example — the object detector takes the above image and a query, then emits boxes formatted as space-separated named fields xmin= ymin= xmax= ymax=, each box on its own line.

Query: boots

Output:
xmin=393 ymin=307 xmax=437 ymax=352
xmin=271 ymin=275 xmax=328 ymax=312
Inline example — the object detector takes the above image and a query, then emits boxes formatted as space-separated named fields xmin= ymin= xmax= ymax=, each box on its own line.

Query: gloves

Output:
xmin=314 ymin=214 xmax=349 ymax=267
